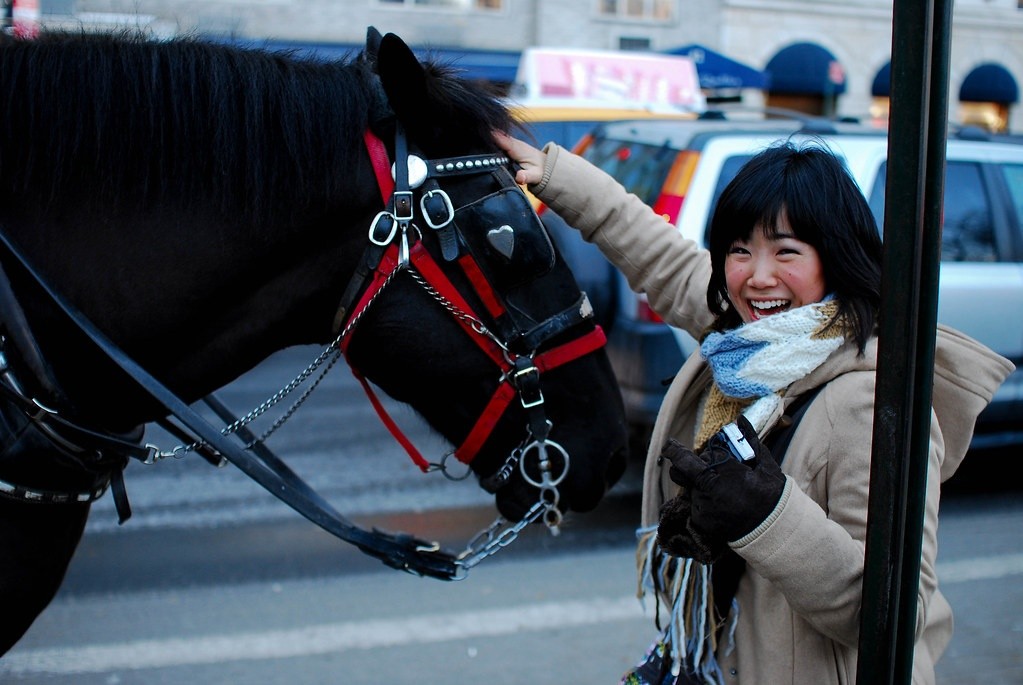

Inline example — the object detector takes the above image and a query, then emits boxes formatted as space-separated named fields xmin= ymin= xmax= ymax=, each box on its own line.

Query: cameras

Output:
xmin=699 ymin=423 xmax=757 ymax=466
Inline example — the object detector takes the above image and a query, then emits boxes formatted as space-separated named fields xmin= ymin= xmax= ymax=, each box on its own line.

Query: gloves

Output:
xmin=660 ymin=412 xmax=786 ymax=542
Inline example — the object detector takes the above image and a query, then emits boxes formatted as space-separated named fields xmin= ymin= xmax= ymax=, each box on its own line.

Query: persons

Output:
xmin=488 ymin=114 xmax=1015 ymax=684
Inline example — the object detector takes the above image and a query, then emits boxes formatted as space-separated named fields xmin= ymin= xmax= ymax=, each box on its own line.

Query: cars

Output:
xmin=526 ymin=104 xmax=1021 ymax=492
xmin=474 ymin=48 xmax=711 ymax=232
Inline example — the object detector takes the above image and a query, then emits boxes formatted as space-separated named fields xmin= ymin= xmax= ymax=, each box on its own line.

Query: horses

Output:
xmin=0 ymin=24 xmax=630 ymax=658
